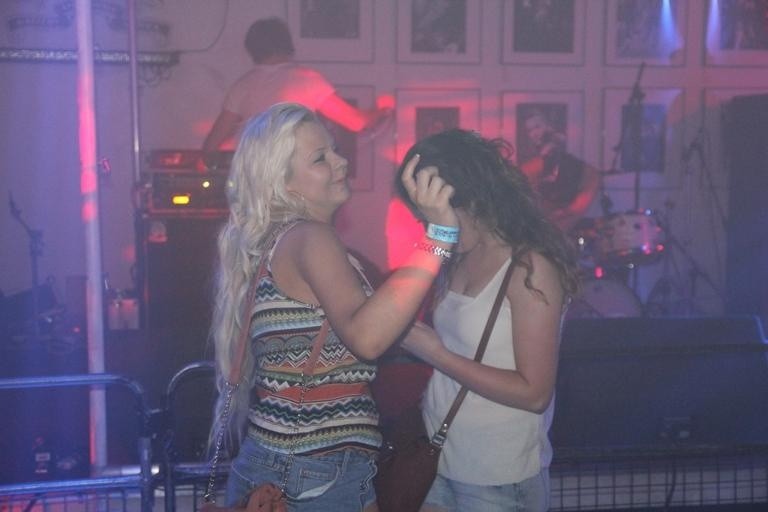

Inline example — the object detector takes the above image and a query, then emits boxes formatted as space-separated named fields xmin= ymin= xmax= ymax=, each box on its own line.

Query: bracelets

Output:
xmin=425 ymin=222 xmax=460 ymax=244
xmin=412 ymin=240 xmax=451 ymax=259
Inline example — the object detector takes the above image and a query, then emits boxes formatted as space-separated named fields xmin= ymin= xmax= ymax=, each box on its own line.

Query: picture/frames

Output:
xmin=496 ymin=87 xmax=590 ymax=180
xmin=390 ymin=85 xmax=482 ymax=176
xmin=597 ymin=84 xmax=682 ymax=195
xmin=599 ymin=0 xmax=682 ymax=69
xmin=496 ymin=1 xmax=587 ymax=68
xmin=283 ymin=1 xmax=375 ymax=66
xmin=392 ymin=2 xmax=484 ymax=66
xmin=316 ymin=81 xmax=376 ymax=196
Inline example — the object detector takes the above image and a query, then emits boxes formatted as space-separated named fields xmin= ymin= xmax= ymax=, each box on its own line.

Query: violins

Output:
xmin=564 ymin=269 xmax=643 ymax=321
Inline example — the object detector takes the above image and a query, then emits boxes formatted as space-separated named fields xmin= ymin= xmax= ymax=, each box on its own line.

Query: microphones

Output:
xmin=599 ymin=166 xmax=628 ymax=176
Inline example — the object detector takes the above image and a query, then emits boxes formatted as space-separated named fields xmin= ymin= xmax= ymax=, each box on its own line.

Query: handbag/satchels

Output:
xmin=198 ymin=482 xmax=288 ymax=512
xmin=370 ymin=405 xmax=444 ymax=512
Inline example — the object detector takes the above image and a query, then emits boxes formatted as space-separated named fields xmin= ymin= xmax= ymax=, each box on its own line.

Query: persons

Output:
xmin=373 ymin=126 xmax=569 ymax=512
xmin=220 ymin=102 xmax=465 ymax=511
xmin=517 ymin=112 xmax=567 ymax=184
xmin=197 ymin=14 xmax=395 ymax=169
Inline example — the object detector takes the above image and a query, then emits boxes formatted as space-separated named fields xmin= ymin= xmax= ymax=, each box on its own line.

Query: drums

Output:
xmin=602 ymin=211 xmax=661 ymax=268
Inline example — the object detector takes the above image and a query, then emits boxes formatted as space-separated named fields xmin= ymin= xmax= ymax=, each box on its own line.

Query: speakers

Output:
xmin=549 ymin=315 xmax=768 ymax=466
xmin=136 ymin=210 xmax=232 ymax=332
